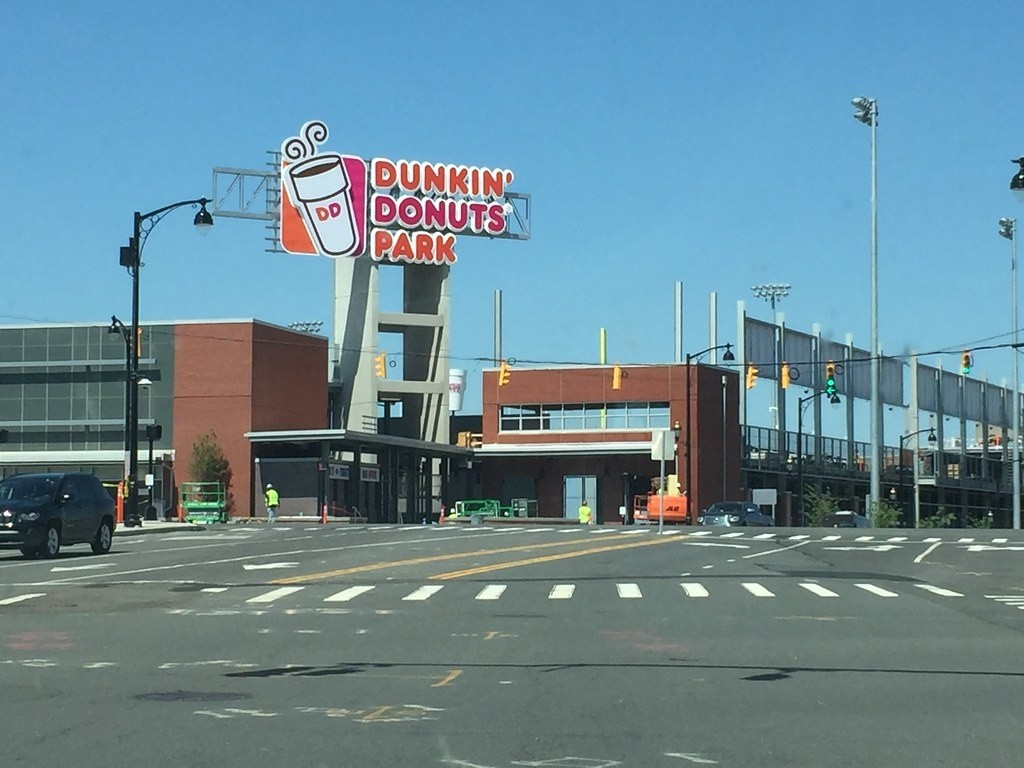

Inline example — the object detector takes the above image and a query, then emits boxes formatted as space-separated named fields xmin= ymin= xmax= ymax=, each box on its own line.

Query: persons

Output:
xmin=264 ymin=483 xmax=280 ymax=525
xmin=578 ymin=500 xmax=592 ymax=526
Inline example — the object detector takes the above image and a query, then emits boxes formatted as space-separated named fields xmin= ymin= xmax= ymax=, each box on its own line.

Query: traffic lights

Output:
xmin=746 ymin=365 xmax=759 ymax=389
xmin=376 ymin=353 xmax=386 ymax=378
xmin=961 ymin=352 xmax=970 ymax=374
xmin=825 ymin=364 xmax=836 ymax=395
xmin=498 ymin=362 xmax=511 ymax=388
xmin=146 ymin=424 xmax=162 ymax=440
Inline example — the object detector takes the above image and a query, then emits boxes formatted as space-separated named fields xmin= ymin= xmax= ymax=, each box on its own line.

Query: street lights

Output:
xmin=798 ymin=391 xmax=840 ymax=527
xmin=673 ymin=420 xmax=681 ymax=481
xmin=899 ymin=427 xmax=937 ymax=527
xmin=684 ymin=342 xmax=735 ymax=525
xmin=850 ymin=96 xmax=881 ymax=505
xmin=751 ymin=282 xmax=790 ymax=324
xmin=999 ymin=217 xmax=1020 ymax=529
xmin=108 ymin=196 xmax=215 ymax=528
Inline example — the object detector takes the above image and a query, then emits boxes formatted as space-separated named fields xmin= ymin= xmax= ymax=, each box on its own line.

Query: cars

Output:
xmin=0 ymin=472 xmax=117 ymax=559
xmin=698 ymin=501 xmax=773 ymax=526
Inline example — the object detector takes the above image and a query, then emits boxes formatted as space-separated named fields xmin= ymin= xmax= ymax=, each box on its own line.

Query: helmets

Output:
xmin=583 ymin=501 xmax=587 ymax=505
xmin=450 ymin=508 xmax=455 ymax=513
xmin=266 ymin=484 xmax=273 ymax=488
